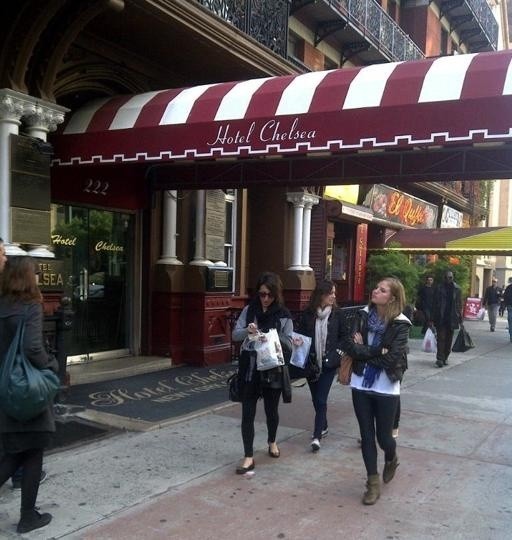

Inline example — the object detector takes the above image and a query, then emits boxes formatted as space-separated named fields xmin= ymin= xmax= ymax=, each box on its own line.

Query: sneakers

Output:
xmin=17 ymin=513 xmax=52 ymax=536
xmin=310 ymin=425 xmax=328 ymax=439
xmin=312 ymin=438 xmax=321 ymax=452
xmin=11 ymin=471 xmax=47 ymax=490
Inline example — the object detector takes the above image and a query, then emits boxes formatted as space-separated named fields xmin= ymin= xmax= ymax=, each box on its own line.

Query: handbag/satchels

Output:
xmin=228 ymin=373 xmax=261 ymax=402
xmin=291 ymin=332 xmax=312 ymax=369
xmin=0 ymin=304 xmax=61 ymax=421
xmin=338 ymin=354 xmax=354 ymax=384
xmin=477 ymin=304 xmax=485 ymax=321
xmin=287 ymin=352 xmax=320 ymax=379
xmin=413 ymin=307 xmax=426 ymax=326
xmin=282 ymin=365 xmax=292 ymax=403
xmin=248 ymin=329 xmax=284 ymax=372
xmin=235 ymin=350 xmax=257 ymax=386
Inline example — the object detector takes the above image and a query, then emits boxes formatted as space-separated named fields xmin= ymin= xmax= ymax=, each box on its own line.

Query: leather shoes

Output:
xmin=392 ymin=427 xmax=398 ymax=438
xmin=236 ymin=457 xmax=255 ymax=474
xmin=267 ymin=439 xmax=280 ymax=457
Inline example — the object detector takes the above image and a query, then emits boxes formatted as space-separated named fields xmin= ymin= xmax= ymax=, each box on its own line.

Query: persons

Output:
xmin=357 ymin=274 xmax=400 ymax=444
xmin=435 ymin=272 xmax=462 ymax=367
xmin=504 ymin=277 xmax=512 ymax=343
xmin=483 ymin=278 xmax=500 ymax=332
xmin=231 ymin=272 xmax=293 ymax=475
xmin=294 ymin=280 xmax=347 ymax=449
xmin=0 ymin=238 xmax=46 ymax=491
xmin=416 ymin=277 xmax=435 ymax=333
xmin=0 ymin=255 xmax=59 ymax=534
xmin=499 ymin=286 xmax=506 ymax=315
xmin=344 ymin=278 xmax=413 ymax=505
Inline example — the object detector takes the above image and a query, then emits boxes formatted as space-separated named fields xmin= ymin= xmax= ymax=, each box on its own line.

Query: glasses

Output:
xmin=259 ymin=292 xmax=274 ymax=298
xmin=444 ymin=277 xmax=452 ymax=280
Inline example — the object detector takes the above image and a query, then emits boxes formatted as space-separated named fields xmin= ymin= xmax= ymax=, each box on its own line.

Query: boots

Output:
xmin=363 ymin=474 xmax=381 ymax=504
xmin=382 ymin=456 xmax=398 ymax=483
